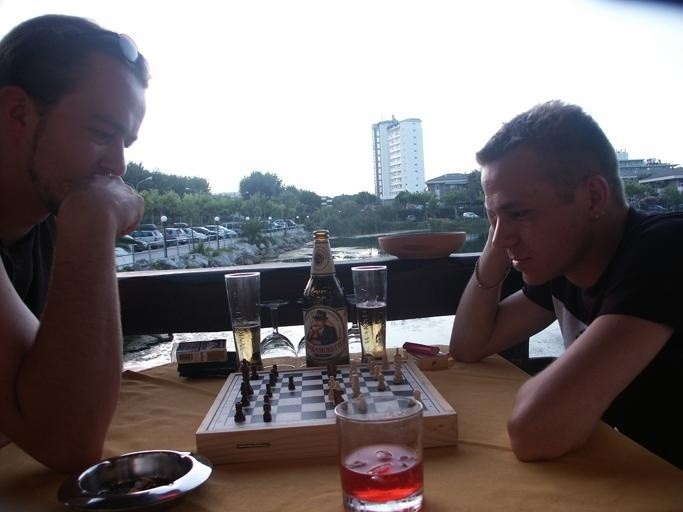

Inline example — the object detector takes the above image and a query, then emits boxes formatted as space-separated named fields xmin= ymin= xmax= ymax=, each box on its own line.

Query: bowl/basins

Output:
xmin=378 ymin=231 xmax=465 ymax=259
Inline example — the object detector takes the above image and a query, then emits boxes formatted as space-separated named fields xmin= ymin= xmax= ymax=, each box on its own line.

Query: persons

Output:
xmin=0 ymin=14 xmax=151 ymax=491
xmin=447 ymin=98 xmax=683 ymax=473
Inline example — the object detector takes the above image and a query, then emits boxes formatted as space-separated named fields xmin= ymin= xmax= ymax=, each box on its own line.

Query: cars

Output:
xmin=407 ymin=214 xmax=417 ymax=221
xmin=116 ymin=219 xmax=301 ymax=252
xmin=463 ymin=211 xmax=479 ymax=218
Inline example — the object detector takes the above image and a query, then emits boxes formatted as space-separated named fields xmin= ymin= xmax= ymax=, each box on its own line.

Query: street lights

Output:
xmin=245 ymin=216 xmax=251 ymax=243
xmin=160 ymin=216 xmax=169 ymax=257
xmin=215 ymin=216 xmax=220 ymax=250
xmin=296 ymin=214 xmax=300 ymax=235
xmin=268 ymin=216 xmax=273 ymax=239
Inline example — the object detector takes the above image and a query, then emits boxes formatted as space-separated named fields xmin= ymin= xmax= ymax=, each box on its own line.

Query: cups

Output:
xmin=334 ymin=395 xmax=425 ymax=512
xmin=223 ymin=272 xmax=262 ymax=371
xmin=350 ymin=266 xmax=387 ymax=360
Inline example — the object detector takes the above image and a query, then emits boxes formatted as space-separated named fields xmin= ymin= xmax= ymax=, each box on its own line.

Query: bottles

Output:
xmin=303 ymin=229 xmax=351 ymax=369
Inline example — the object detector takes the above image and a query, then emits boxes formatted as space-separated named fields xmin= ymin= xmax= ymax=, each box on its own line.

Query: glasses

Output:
xmin=73 ymin=29 xmax=152 ymax=84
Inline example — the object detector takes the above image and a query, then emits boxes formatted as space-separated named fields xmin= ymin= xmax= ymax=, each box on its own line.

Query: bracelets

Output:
xmin=472 ymin=256 xmax=512 ymax=290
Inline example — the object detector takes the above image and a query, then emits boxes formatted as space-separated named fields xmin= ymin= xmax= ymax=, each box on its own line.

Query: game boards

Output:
xmin=196 ymin=358 xmax=459 ymax=466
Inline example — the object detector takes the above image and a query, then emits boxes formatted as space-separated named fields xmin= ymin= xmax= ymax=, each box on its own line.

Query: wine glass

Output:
xmin=255 ymin=297 xmax=306 ymax=373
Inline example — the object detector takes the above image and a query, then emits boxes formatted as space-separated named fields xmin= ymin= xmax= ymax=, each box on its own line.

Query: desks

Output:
xmin=1 ymin=344 xmax=683 ymax=512
